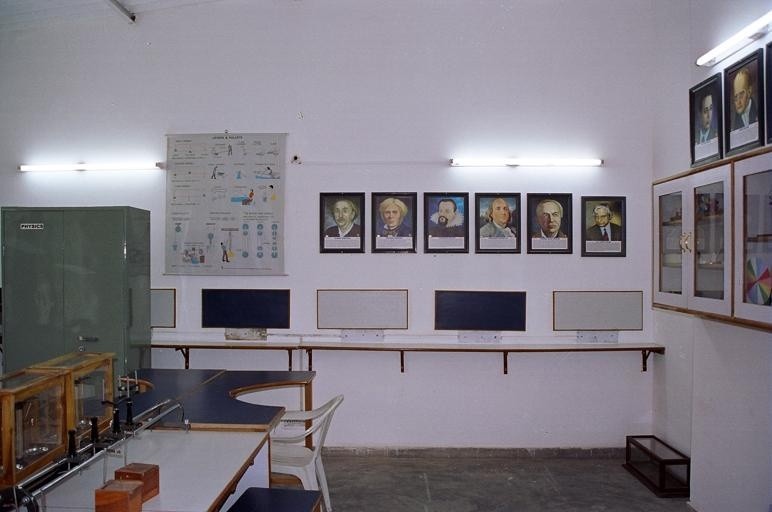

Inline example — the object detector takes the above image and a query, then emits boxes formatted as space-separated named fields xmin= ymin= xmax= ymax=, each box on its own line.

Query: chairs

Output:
xmin=269 ymin=393 xmax=347 ymax=511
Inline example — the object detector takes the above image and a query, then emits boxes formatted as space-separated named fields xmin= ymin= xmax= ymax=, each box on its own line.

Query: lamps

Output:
xmin=696 ymin=8 xmax=772 ymax=69
xmin=18 ymin=162 xmax=164 ymax=173
xmin=446 ymin=159 xmax=605 ymax=168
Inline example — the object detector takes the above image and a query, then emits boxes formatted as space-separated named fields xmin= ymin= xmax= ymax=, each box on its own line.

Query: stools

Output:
xmin=228 ymin=486 xmax=325 ymax=512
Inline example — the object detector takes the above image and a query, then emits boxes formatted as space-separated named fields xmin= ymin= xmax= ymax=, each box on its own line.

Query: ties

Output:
xmin=744 ymin=112 xmax=749 ymax=126
xmin=502 ymin=228 xmax=512 ymax=237
xmin=384 ymin=228 xmax=399 ymax=237
xmin=701 ymin=136 xmax=706 ymax=142
xmin=602 ymin=228 xmax=609 ymax=241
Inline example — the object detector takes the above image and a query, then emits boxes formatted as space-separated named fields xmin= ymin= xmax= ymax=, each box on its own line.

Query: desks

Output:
xmin=2 ymin=369 xmax=317 ymax=512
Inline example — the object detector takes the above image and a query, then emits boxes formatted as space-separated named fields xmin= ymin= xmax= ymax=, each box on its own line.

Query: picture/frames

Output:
xmin=371 ymin=192 xmax=418 ymax=254
xmin=473 ymin=192 xmax=521 ymax=255
xmin=687 ymin=72 xmax=723 ymax=169
xmin=580 ymin=195 xmax=627 ymax=257
xmin=423 ymin=192 xmax=470 ymax=255
xmin=319 ymin=191 xmax=366 ymax=255
xmin=526 ymin=192 xmax=574 ymax=255
xmin=723 ymin=48 xmax=765 ymax=157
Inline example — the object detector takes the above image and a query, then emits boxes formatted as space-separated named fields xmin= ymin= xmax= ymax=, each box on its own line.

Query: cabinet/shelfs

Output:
xmin=0 ymin=206 xmax=152 ymax=390
xmin=652 ymin=148 xmax=772 ymax=333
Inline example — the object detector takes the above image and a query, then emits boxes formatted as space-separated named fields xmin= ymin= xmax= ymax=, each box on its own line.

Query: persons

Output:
xmin=585 ymin=202 xmax=621 ymax=241
xmin=530 ymin=200 xmax=569 ymax=238
xmin=325 ymin=198 xmax=360 ymax=237
xmin=479 ymin=198 xmax=516 ymax=238
xmin=694 ymin=88 xmax=718 ymax=146
xmin=376 ymin=198 xmax=411 ymax=237
xmin=427 ymin=199 xmax=464 ymax=236
xmin=733 ymin=69 xmax=757 ymax=130
